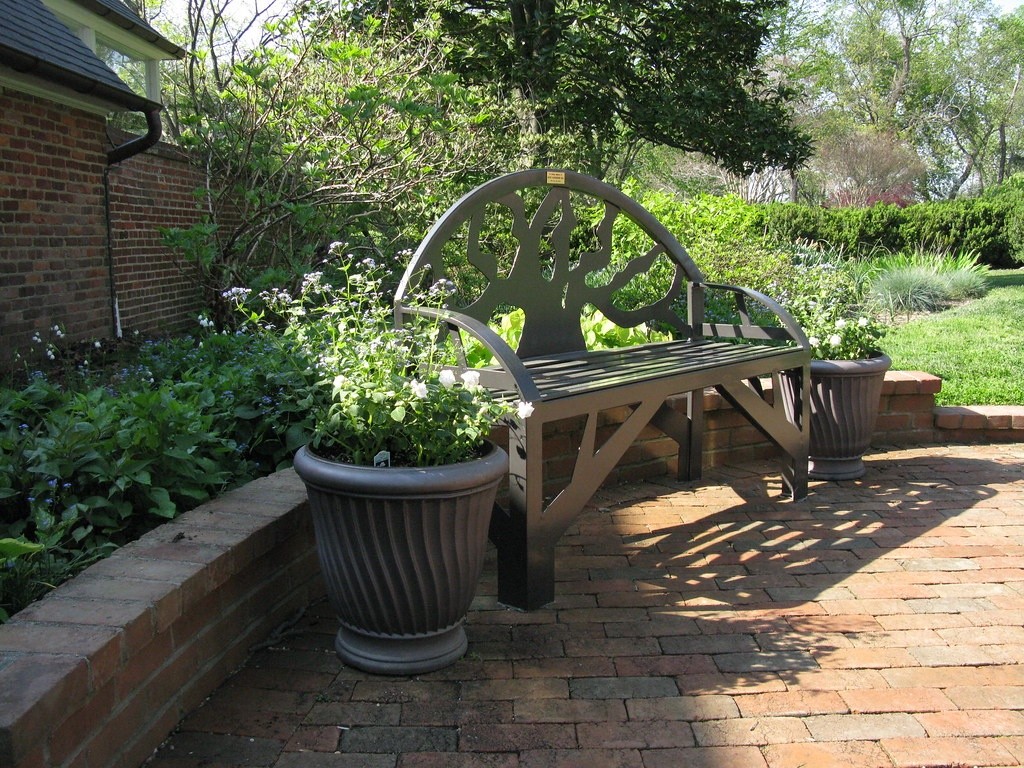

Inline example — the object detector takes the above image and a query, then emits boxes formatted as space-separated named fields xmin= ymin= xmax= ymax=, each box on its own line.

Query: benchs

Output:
xmin=393 ymin=167 xmax=811 ymax=612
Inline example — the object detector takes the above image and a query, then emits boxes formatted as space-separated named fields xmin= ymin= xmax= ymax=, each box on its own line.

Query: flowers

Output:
xmin=764 ymin=239 xmax=887 ymax=361
xmin=221 ymin=241 xmax=535 ymax=467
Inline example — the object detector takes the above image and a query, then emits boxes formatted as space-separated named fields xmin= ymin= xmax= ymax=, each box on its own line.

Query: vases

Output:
xmin=777 ymin=352 xmax=892 ymax=479
xmin=294 ymin=434 xmax=511 ymax=676
